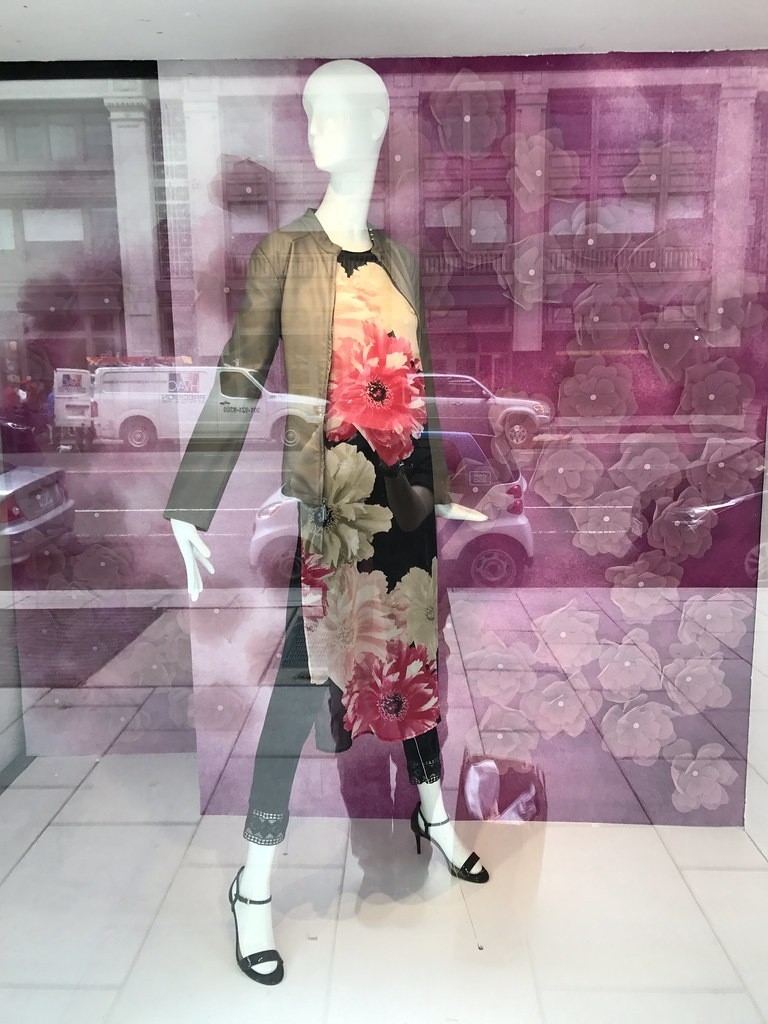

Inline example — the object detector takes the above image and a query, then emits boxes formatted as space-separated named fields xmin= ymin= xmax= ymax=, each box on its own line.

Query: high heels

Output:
xmin=409 ymin=802 xmax=490 ymax=884
xmin=227 ymin=865 xmax=284 ymax=987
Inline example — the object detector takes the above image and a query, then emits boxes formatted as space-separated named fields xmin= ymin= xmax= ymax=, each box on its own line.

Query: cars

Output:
xmin=403 ymin=373 xmax=552 ymax=447
xmin=0 ymin=457 xmax=77 ymax=571
xmin=251 ymin=431 xmax=535 ymax=587
xmin=0 ymin=417 xmax=36 ymax=451
xmin=625 ymin=443 xmax=767 ymax=587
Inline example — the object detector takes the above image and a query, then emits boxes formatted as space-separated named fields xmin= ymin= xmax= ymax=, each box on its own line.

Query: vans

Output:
xmin=50 ymin=368 xmax=333 ymax=446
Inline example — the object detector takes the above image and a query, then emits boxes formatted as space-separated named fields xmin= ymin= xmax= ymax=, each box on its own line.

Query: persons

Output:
xmin=163 ymin=58 xmax=490 ymax=984
xmin=4 ymin=379 xmax=95 ymax=452
